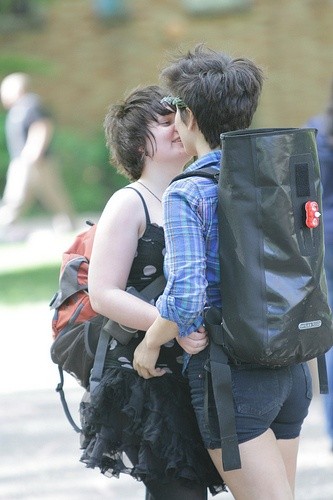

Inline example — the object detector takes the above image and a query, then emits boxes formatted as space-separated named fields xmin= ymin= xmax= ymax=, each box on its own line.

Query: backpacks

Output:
xmin=170 ymin=126 xmax=333 ymax=370
xmin=48 ymin=222 xmax=105 ymax=390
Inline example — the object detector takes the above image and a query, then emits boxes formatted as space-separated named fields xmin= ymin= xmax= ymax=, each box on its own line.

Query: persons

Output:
xmin=79 ymin=44 xmax=313 ymax=500
xmin=1 ymin=71 xmax=79 ymax=244
